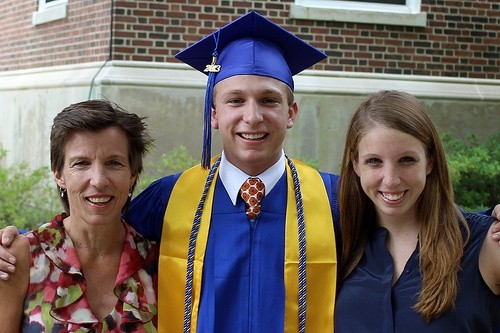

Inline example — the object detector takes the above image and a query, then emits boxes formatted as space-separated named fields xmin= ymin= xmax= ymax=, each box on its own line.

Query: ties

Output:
xmin=240 ymin=177 xmax=264 ymax=219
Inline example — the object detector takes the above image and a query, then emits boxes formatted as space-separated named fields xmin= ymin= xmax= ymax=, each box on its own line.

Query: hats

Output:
xmin=174 ymin=10 xmax=328 ymax=171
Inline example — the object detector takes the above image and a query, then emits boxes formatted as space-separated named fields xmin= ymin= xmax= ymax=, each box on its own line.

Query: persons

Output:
xmin=334 ymin=90 xmax=500 ymax=333
xmin=0 ymin=99 xmax=159 ymax=333
xmin=0 ymin=11 xmax=500 ymax=333
